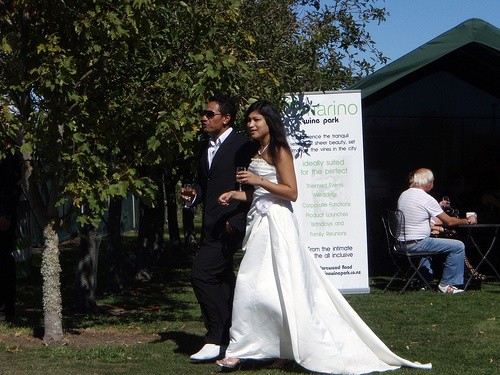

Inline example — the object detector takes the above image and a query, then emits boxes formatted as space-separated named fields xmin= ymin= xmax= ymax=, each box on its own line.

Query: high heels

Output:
xmin=217 ymin=358 xmax=246 ymax=370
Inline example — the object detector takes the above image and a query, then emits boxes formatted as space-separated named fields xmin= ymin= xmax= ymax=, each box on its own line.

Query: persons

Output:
xmin=216 ymin=100 xmax=432 ymax=375
xmin=396 ymin=168 xmax=477 ymax=293
xmin=181 ymin=203 xmax=197 ymax=243
xmin=178 ymin=96 xmax=259 ymax=361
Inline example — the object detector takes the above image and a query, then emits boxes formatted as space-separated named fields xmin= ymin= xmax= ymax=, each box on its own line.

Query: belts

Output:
xmin=398 ymin=239 xmax=423 ymax=245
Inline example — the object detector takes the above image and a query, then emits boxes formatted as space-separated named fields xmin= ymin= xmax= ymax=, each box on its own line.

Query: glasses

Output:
xmin=202 ymin=109 xmax=220 ymax=118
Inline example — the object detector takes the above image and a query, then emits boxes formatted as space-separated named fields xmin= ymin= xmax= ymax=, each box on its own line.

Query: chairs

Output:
xmin=381 ymin=208 xmax=440 ymax=296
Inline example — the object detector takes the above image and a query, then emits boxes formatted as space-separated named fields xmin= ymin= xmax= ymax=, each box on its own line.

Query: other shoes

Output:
xmin=438 ymin=284 xmax=464 ymax=294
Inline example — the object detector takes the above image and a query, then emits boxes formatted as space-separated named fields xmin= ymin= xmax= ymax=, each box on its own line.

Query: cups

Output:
xmin=466 ymin=212 xmax=477 ymax=225
xmin=443 ymin=197 xmax=450 ymax=209
xmin=183 ymin=184 xmax=197 ymax=208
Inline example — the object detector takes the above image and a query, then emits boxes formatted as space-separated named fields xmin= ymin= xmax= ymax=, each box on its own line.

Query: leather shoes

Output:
xmin=190 ymin=344 xmax=227 ymax=360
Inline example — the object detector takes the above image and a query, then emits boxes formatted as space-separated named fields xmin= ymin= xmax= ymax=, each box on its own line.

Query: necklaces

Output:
xmin=258 ymin=143 xmax=269 ymax=155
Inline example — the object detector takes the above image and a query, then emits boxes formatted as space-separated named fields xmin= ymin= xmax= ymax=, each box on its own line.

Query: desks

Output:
xmin=442 ymin=224 xmax=500 ymax=291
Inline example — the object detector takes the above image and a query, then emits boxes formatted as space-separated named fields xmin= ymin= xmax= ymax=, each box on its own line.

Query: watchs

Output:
xmin=467 ymin=218 xmax=471 ymax=224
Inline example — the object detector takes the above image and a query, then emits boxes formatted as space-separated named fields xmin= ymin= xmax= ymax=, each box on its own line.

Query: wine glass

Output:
xmin=237 ymin=167 xmax=246 ymax=192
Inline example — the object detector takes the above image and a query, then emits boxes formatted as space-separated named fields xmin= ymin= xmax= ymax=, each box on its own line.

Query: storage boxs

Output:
xmin=455 ymin=272 xmax=481 ymax=290
